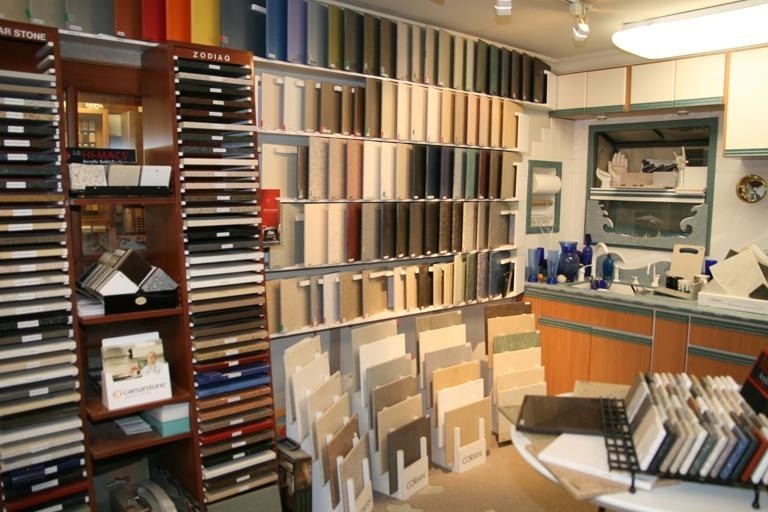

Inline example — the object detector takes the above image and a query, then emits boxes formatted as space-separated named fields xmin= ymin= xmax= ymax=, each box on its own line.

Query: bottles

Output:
xmin=604 ymin=254 xmax=613 ymax=281
xmin=581 ymin=234 xmax=592 ymax=277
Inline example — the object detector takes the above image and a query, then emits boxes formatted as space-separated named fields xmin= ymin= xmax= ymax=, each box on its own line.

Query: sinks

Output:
xmin=571 ymin=280 xmax=653 ymax=297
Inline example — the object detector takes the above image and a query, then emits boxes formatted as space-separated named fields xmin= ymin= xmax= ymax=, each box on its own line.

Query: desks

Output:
xmin=511 ymin=390 xmax=768 ymax=511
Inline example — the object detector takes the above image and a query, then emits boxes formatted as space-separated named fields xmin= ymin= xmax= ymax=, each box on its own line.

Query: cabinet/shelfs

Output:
xmin=523 ymin=288 xmax=768 ymax=399
xmin=628 ymin=51 xmax=728 ymax=110
xmin=548 ymin=62 xmax=631 ymax=117
xmin=722 ymin=44 xmax=768 ymax=159
xmin=0 ymin=19 xmax=282 ymax=511
xmin=0 ymin=0 xmax=558 ymax=512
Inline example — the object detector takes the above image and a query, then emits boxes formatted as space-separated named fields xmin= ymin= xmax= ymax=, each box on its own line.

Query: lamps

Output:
xmin=609 ymin=0 xmax=767 ymax=63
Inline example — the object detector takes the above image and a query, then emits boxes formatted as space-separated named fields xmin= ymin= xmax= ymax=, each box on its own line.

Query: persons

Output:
xmin=608 ymin=153 xmax=628 ymax=187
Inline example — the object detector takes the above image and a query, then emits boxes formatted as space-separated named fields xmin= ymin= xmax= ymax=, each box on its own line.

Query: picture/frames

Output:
xmin=735 ymin=174 xmax=768 ymax=204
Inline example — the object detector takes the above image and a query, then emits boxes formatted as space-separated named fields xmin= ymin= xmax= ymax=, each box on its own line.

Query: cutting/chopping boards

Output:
xmin=669 ymin=244 xmax=705 ymax=281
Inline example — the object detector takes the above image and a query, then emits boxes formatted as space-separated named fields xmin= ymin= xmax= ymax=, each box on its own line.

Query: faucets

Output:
xmin=647 ymin=262 xmax=656 ymax=283
xmin=591 ymin=242 xmax=609 ymax=279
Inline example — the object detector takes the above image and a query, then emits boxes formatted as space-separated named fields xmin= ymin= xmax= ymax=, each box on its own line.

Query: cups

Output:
xmin=547 ymin=250 xmax=558 ymax=285
xmin=527 ymin=248 xmax=541 ymax=282
